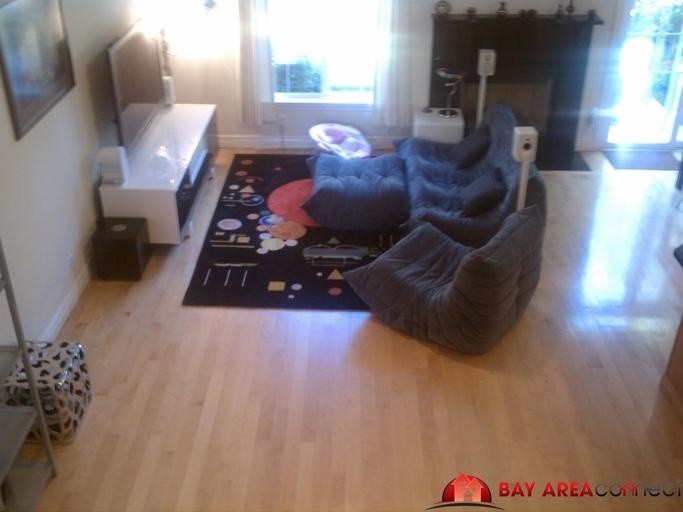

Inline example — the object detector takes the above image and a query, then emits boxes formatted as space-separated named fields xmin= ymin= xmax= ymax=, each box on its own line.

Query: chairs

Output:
xmin=343 ymin=204 xmax=547 ymax=355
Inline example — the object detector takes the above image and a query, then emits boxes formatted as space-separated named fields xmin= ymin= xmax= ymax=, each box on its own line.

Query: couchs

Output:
xmin=393 ymin=101 xmax=552 ymax=248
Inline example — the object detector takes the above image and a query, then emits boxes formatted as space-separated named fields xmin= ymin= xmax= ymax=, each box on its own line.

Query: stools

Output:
xmin=93 ymin=217 xmax=150 ymax=279
xmin=0 ymin=337 xmax=93 ymax=444
xmin=300 ymin=152 xmax=401 ymax=235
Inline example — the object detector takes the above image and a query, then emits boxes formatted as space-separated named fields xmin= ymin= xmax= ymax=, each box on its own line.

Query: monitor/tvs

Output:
xmin=105 ymin=20 xmax=167 ymax=157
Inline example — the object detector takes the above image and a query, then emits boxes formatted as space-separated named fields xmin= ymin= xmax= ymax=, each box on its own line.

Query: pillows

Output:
xmin=456 ymin=168 xmax=504 ymax=213
xmin=458 ymin=125 xmax=491 ymax=162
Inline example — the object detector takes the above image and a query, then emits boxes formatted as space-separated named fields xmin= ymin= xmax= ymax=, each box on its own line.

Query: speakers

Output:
xmin=478 ymin=50 xmax=496 ymax=76
xmin=513 ymin=127 xmax=539 ymax=161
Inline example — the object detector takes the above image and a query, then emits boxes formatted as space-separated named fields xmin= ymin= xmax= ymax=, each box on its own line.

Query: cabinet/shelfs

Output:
xmin=0 ymin=238 xmax=58 ymax=512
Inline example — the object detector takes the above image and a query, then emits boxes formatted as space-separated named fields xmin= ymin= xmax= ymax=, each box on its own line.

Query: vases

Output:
xmin=98 ymin=102 xmax=221 ymax=247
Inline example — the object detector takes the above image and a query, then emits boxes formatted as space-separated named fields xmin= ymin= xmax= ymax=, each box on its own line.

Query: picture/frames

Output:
xmin=0 ymin=0 xmax=77 ymax=140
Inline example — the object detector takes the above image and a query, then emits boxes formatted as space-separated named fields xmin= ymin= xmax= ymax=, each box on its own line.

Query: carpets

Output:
xmin=604 ymin=149 xmax=682 ymax=170
xmin=553 ymin=153 xmax=591 ymax=172
xmin=179 ymin=153 xmax=408 ymax=311
